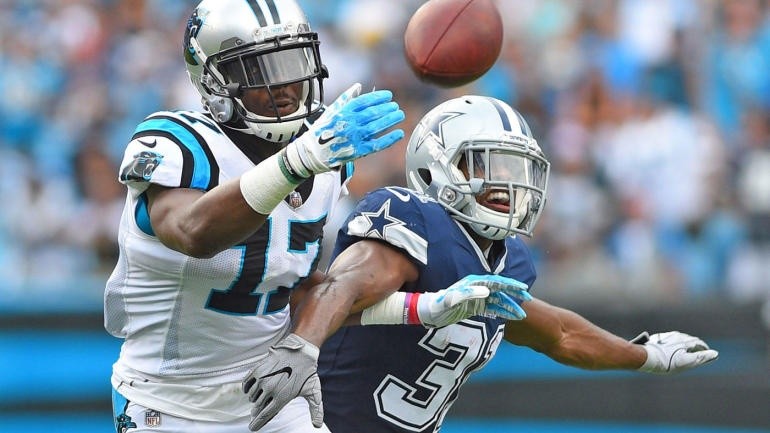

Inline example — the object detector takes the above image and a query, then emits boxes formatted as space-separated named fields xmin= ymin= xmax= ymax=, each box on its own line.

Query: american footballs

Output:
xmin=402 ymin=0 xmax=504 ymax=89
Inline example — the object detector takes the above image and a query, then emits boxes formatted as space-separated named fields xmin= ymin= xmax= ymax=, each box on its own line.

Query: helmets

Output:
xmin=404 ymin=96 xmax=550 ymax=240
xmin=183 ymin=0 xmax=329 ymax=142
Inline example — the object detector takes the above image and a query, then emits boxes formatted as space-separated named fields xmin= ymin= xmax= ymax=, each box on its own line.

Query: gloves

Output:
xmin=629 ymin=331 xmax=720 ymax=372
xmin=295 ymin=83 xmax=406 ymax=175
xmin=243 ymin=335 xmax=325 ymax=431
xmin=417 ymin=274 xmax=532 ymax=329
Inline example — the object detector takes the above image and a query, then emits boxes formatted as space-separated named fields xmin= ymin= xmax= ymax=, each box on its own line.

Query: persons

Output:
xmin=514 ymin=0 xmax=770 ymax=294
xmin=1 ymin=0 xmax=553 ymax=297
xmin=105 ymin=0 xmax=535 ymax=433
xmin=241 ymin=95 xmax=720 ymax=433
